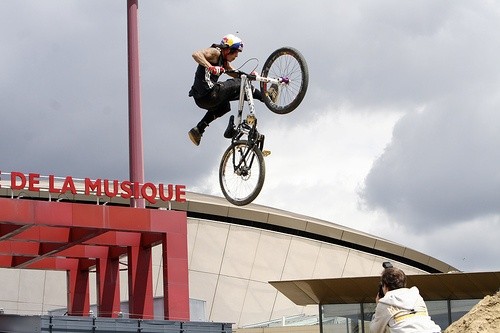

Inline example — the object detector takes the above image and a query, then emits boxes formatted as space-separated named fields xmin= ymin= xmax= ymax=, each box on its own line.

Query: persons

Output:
xmin=188 ymin=33 xmax=280 ymax=147
xmin=369 ymin=267 xmax=443 ymax=333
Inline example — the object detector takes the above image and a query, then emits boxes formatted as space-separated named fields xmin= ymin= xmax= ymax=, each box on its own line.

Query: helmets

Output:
xmin=220 ymin=34 xmax=243 ymax=52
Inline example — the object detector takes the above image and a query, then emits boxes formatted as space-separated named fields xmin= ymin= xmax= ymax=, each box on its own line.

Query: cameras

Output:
xmin=378 ymin=261 xmax=393 ymax=298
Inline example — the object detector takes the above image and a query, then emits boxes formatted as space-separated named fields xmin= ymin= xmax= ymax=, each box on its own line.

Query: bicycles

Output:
xmin=209 ymin=47 xmax=309 ymax=206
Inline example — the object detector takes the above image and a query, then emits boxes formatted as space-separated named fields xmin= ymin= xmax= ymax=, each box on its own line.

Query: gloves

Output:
xmin=249 ymin=71 xmax=259 ymax=81
xmin=208 ymin=66 xmax=225 ymax=76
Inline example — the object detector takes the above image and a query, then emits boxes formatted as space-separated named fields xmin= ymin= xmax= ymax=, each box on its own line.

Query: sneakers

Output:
xmin=267 ymin=83 xmax=277 ymax=106
xmin=188 ymin=127 xmax=205 ymax=147
